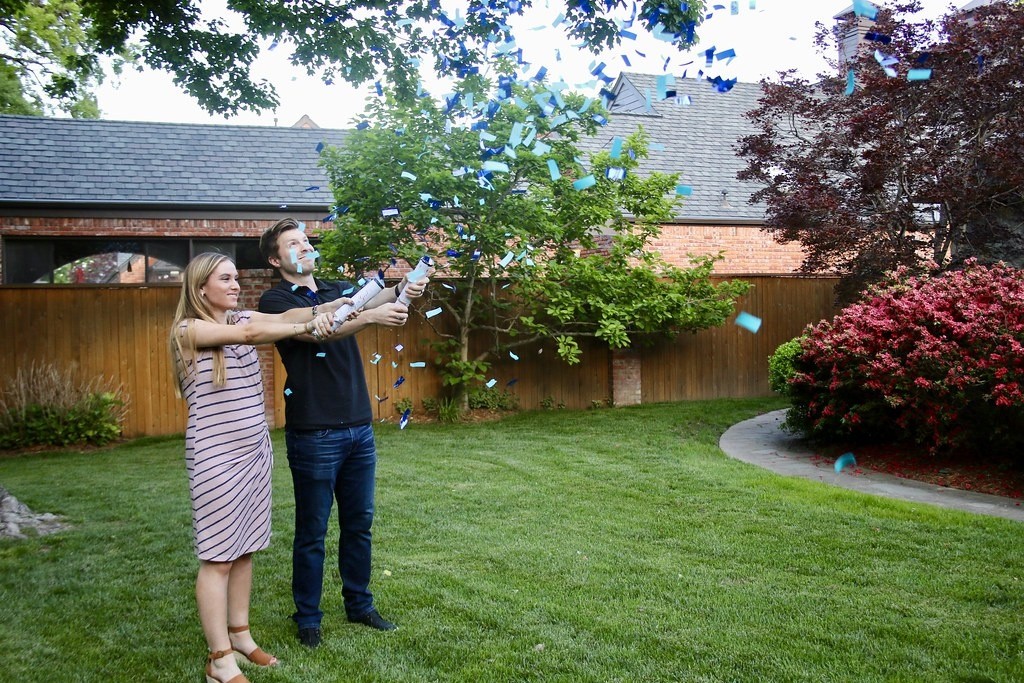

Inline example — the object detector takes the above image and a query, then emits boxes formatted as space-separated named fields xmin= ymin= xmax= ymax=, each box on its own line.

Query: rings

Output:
xmin=396 ymin=313 xmax=399 ymax=320
xmin=421 ymin=289 xmax=425 ymax=293
xmin=311 ymin=304 xmax=318 ymax=317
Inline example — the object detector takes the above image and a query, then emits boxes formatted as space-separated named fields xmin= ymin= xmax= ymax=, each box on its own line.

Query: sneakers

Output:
xmin=357 ymin=610 xmax=396 ymax=630
xmin=298 ymin=627 xmax=323 ymax=648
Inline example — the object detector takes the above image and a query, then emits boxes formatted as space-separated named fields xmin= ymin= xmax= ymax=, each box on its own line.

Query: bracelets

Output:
xmin=293 ymin=321 xmax=299 ymax=336
xmin=306 ymin=321 xmax=313 ymax=335
xmin=394 ymin=283 xmax=401 ymax=298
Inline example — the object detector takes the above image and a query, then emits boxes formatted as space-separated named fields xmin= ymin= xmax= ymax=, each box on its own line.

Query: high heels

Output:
xmin=227 ymin=624 xmax=278 ymax=669
xmin=205 ymin=648 xmax=251 ymax=683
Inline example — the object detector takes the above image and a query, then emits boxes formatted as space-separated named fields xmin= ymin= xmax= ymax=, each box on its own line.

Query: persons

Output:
xmin=258 ymin=217 xmax=430 ymax=647
xmin=167 ymin=251 xmax=365 ymax=683
